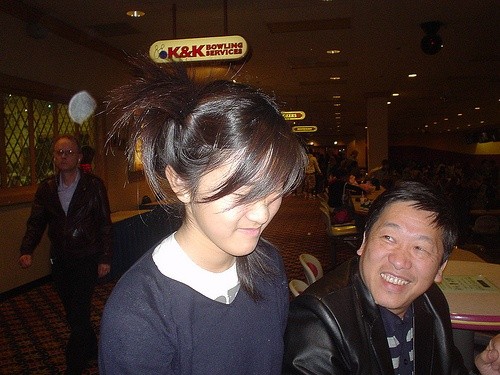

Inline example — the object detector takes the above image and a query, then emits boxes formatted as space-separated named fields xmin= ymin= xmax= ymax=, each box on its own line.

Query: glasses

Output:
xmin=54 ymin=149 xmax=80 ymax=156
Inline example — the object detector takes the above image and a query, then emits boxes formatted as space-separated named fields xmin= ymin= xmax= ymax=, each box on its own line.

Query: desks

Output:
xmin=350 ymin=182 xmax=385 ymax=229
xmin=104 ymin=193 xmax=183 ymax=281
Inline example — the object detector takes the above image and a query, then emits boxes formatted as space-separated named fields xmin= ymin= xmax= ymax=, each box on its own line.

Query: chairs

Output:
xmin=289 ymin=185 xmax=361 ymax=296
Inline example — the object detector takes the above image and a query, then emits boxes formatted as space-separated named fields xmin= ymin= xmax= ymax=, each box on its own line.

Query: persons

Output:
xmin=17 ymin=134 xmax=113 ymax=375
xmin=282 ymin=180 xmax=500 ymax=375
xmin=90 ymin=39 xmax=312 ymax=375
xmin=289 ymin=148 xmax=401 ymax=208
